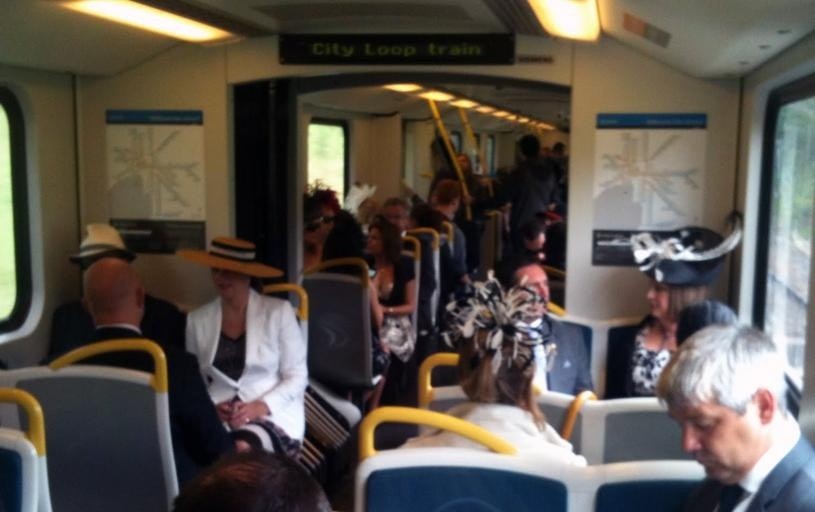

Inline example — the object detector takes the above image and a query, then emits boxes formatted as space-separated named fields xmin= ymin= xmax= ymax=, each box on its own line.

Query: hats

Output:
xmin=632 ymin=225 xmax=729 ymax=285
xmin=178 ymin=236 xmax=285 ymax=278
xmin=70 ymin=223 xmax=133 ymax=263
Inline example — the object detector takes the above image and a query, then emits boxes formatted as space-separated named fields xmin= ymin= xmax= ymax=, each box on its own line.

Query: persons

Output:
xmin=606 ymin=226 xmax=734 ymax=401
xmin=514 ymin=264 xmax=596 ymax=395
xmin=41 ymin=223 xmax=307 ymax=481
xmin=304 ymin=133 xmax=569 ymax=378
xmin=653 ymin=319 xmax=815 ymax=512
xmin=173 ymin=454 xmax=332 ymax=511
xmin=403 ymin=279 xmax=586 ymax=470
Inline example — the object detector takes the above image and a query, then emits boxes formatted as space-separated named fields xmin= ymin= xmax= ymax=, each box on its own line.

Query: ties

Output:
xmin=717 ymin=485 xmax=742 ymax=512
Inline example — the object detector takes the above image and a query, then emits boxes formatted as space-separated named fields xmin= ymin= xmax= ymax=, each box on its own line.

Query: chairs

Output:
xmin=0 ymin=186 xmax=813 ymax=512
